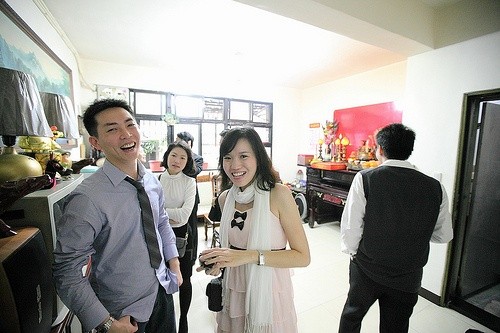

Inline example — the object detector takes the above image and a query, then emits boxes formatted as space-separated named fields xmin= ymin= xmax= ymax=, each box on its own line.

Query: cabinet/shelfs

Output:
xmin=297 ymin=164 xmax=358 ymax=228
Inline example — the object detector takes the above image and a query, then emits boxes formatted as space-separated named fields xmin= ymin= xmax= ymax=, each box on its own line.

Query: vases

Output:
xmin=326 ymin=144 xmax=331 ymax=154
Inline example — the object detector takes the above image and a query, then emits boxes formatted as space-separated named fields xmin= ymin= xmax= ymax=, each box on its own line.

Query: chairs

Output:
xmin=204 ymin=173 xmax=219 ymax=248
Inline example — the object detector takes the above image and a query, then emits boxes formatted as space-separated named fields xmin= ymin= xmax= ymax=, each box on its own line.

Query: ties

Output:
xmin=123 ymin=176 xmax=162 ymax=270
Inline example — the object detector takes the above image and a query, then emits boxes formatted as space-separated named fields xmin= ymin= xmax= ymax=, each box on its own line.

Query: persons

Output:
xmin=196 ymin=126 xmax=311 ymax=333
xmin=340 ymin=123 xmax=454 ymax=333
xmin=51 ymin=99 xmax=203 ymax=333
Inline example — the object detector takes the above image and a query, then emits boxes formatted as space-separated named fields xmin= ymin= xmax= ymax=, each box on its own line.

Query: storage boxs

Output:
xmin=297 ymin=154 xmax=314 ymax=165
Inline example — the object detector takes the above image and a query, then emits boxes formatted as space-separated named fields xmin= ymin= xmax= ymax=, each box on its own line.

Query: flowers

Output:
xmin=321 ymin=119 xmax=339 ymax=145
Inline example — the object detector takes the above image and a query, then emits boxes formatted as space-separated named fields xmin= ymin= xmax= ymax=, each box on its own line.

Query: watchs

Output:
xmin=256 ymin=249 xmax=266 ymax=266
xmin=99 ymin=316 xmax=115 ymax=333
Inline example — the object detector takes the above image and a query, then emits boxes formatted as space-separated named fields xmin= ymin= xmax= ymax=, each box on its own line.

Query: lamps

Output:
xmin=0 ymin=67 xmax=76 ymax=182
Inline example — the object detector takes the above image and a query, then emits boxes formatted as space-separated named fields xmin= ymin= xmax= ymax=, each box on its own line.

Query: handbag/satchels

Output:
xmin=206 ymin=277 xmax=224 ymax=312
xmin=175 ymin=236 xmax=189 ymax=259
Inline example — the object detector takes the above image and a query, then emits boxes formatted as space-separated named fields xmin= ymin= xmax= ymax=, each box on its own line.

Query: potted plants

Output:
xmin=140 ymin=136 xmax=166 ymax=171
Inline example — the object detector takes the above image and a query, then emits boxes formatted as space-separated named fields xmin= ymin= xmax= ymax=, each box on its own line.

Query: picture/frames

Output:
xmin=0 ymin=0 xmax=74 ymax=113
